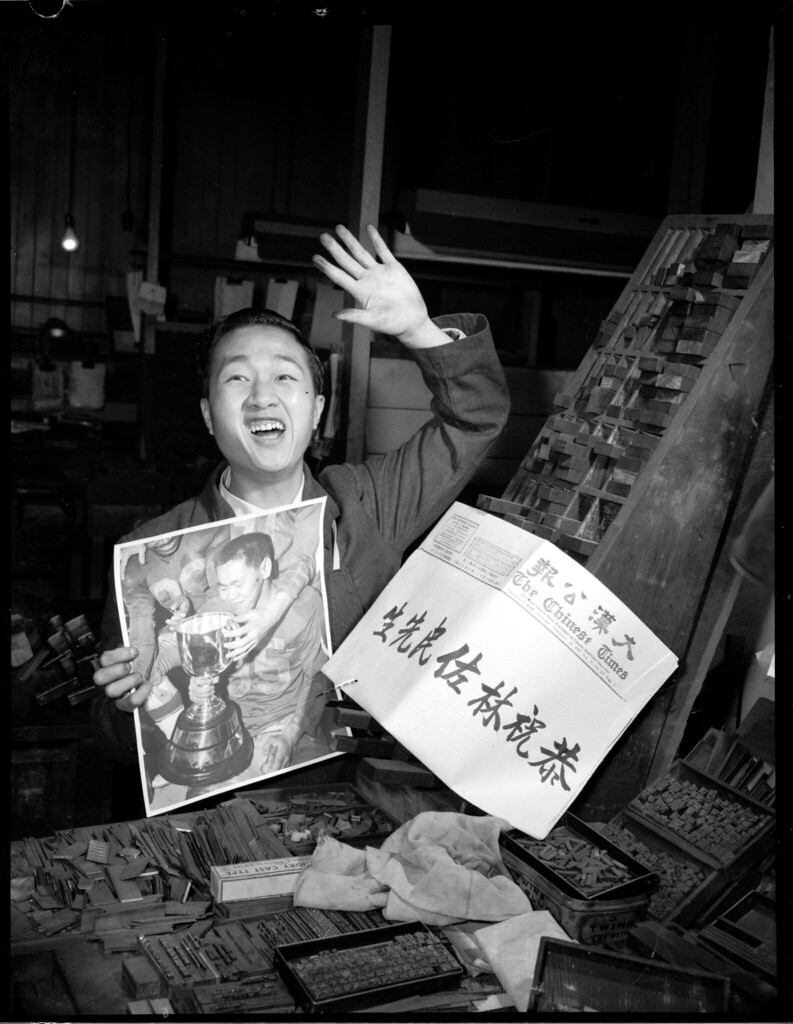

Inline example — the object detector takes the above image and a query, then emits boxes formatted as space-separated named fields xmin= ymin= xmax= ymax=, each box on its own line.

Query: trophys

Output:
xmin=159 ymin=612 xmax=254 ymax=785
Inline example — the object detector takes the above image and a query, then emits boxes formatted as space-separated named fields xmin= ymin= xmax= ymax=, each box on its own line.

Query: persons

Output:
xmin=117 ymin=505 xmax=321 ymax=794
xmin=86 ymin=223 xmax=512 ymax=806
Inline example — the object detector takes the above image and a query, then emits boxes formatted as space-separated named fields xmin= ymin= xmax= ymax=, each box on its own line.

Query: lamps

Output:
xmin=61 ymin=214 xmax=82 ymax=253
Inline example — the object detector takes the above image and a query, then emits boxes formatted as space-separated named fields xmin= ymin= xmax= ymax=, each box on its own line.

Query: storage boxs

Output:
xmin=498 ymin=845 xmax=649 ymax=950
xmin=243 ymin=216 xmax=348 ymax=267
xmin=376 ymin=184 xmax=667 ymax=275
